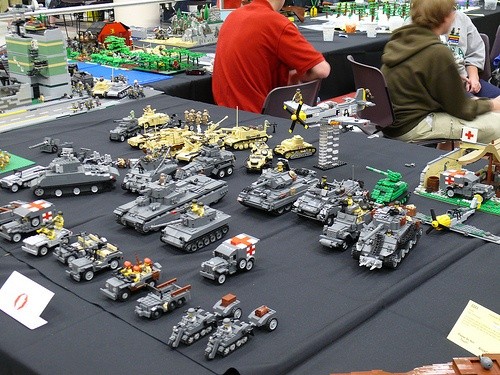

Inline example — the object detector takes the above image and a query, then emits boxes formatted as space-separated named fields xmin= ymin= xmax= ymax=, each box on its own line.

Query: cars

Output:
xmin=21 ymin=225 xmax=73 ymax=256
xmin=100 ymin=261 xmax=191 ymax=320
xmin=186 ymin=67 xmax=207 ymax=74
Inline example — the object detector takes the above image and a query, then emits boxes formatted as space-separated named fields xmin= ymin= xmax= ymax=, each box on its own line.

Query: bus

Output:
xmin=91 ymin=80 xmax=133 ymax=98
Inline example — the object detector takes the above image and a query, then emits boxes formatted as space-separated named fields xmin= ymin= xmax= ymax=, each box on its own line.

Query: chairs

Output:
xmin=262 ymin=79 xmax=320 ymax=119
xmin=490 ymin=25 xmax=500 ymax=60
xmin=479 ymin=34 xmax=490 ymax=81
xmin=347 ymin=55 xmax=455 ymax=150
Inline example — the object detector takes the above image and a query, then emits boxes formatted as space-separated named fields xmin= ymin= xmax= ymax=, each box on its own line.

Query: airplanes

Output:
xmin=284 ymin=88 xmax=378 ymax=135
xmin=414 ymin=198 xmax=500 ymax=245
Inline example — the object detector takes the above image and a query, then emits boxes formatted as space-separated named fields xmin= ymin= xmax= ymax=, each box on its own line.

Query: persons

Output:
xmin=186 ymin=308 xmax=197 ymax=324
xmin=277 ymin=162 xmax=284 ymax=172
xmin=120 ymin=261 xmax=140 ymax=284
xmin=190 ymin=199 xmax=205 ymax=218
xmin=70 ymin=82 xmax=90 ymax=96
xmin=438 ymin=9 xmax=500 ymax=102
xmin=51 ymin=211 xmax=65 ymax=228
xmin=156 ymin=174 xmax=166 ymax=186
xmin=221 ymin=318 xmax=232 ymax=337
xmin=139 ymin=258 xmax=152 ymax=273
xmin=146 ymin=106 xmax=152 ymax=113
xmin=376 ymin=0 xmax=500 ymax=145
xmin=183 ymin=109 xmax=210 ymax=124
xmin=134 ymin=80 xmax=140 ymax=89
xmin=73 ymin=67 xmax=79 ymax=76
xmin=212 ymin=0 xmax=330 ymax=116
xmin=145 ymin=148 xmax=160 ymax=162
xmin=320 ymin=175 xmax=402 ymax=237
xmin=72 ymin=96 xmax=101 ymax=113
xmin=126 ymin=110 xmax=135 ymax=119
xmin=0 ymin=149 xmax=10 ymax=170
xmin=36 ymin=223 xmax=56 ymax=240
xmin=292 ymin=88 xmax=303 ymax=103
xmin=98 ymin=75 xmax=126 ymax=83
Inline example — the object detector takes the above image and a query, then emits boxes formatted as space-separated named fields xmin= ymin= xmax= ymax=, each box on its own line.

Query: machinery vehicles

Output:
xmin=0 ymin=137 xmax=119 ymax=197
xmin=121 ymin=147 xmax=179 ymax=195
xmin=151 ymin=204 xmax=231 ymax=252
xmin=272 ymin=135 xmax=316 ymax=159
xmin=52 ymin=231 xmax=123 ymax=282
xmin=113 ymin=174 xmax=229 ymax=233
xmin=291 ymin=166 xmax=424 ymax=272
xmin=109 ymin=109 xmax=273 ymax=178
xmin=237 ymin=158 xmax=320 ymax=215
xmin=168 ymin=293 xmax=278 ymax=361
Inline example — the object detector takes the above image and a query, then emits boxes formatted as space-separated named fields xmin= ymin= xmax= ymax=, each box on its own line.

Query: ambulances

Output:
xmin=440 ymin=170 xmax=496 ymax=199
xmin=0 ymin=199 xmax=57 ymax=243
xmin=199 ymin=233 xmax=260 ymax=284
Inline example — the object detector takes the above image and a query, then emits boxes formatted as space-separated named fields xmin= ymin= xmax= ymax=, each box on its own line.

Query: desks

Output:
xmin=0 ymin=0 xmax=500 ymax=375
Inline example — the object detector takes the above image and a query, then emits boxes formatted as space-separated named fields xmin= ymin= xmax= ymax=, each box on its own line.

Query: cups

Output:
xmin=322 ymin=23 xmax=335 ymax=41
xmin=366 ymin=23 xmax=378 ymax=38
